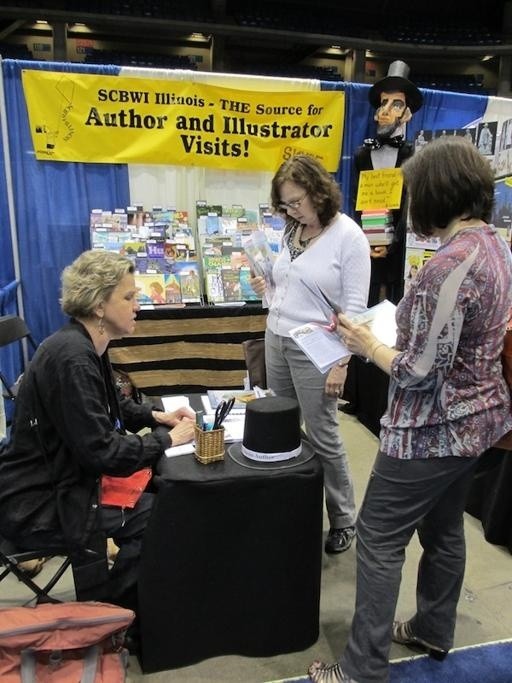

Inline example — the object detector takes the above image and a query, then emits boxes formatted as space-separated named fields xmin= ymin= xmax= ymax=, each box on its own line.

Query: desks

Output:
xmin=137 ymin=393 xmax=323 ymax=675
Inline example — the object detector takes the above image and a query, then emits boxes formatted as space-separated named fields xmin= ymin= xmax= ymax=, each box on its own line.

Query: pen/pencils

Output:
xmin=196 ymin=410 xmax=206 ymax=432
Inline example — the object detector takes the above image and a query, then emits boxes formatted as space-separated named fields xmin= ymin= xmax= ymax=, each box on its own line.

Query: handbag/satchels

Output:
xmin=99 ymin=464 xmax=152 ymax=509
xmin=110 ymin=367 xmax=142 ymax=402
xmin=242 ymin=339 xmax=267 ymax=390
xmin=0 ymin=601 xmax=136 ymax=683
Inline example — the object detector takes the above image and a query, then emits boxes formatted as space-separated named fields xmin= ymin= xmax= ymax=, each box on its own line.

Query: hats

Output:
xmin=369 ymin=59 xmax=424 ymax=110
xmin=228 ymin=396 xmax=315 ymax=471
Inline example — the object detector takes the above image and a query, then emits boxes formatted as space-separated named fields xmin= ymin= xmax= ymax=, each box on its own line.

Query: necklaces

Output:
xmin=299 ymin=227 xmax=324 ymax=248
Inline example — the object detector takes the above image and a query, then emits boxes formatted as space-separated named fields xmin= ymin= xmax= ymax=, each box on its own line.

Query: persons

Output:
xmin=308 ymin=136 xmax=512 ymax=683
xmin=350 ymin=86 xmax=415 ymax=308
xmin=0 ymin=250 xmax=200 ymax=609
xmin=417 ymin=123 xmax=493 ymax=154
xmin=409 ymin=265 xmax=418 ymax=278
xmin=249 ymin=156 xmax=371 ymax=554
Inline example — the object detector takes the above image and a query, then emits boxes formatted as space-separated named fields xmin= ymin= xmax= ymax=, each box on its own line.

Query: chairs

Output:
xmin=0 ymin=315 xmax=38 ymax=399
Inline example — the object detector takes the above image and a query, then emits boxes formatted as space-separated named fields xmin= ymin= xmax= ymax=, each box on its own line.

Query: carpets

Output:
xmin=265 ymin=639 xmax=512 ymax=683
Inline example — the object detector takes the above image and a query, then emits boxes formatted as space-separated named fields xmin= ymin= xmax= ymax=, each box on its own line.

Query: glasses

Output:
xmin=281 ymin=194 xmax=307 ymax=209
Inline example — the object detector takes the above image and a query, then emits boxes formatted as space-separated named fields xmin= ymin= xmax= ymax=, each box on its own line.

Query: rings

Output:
xmin=335 ymin=390 xmax=339 ymax=394
xmin=343 ymin=336 xmax=346 ymax=339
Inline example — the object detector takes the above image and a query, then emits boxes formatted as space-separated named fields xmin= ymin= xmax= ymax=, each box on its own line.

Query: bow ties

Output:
xmin=365 ymin=137 xmax=402 ymax=152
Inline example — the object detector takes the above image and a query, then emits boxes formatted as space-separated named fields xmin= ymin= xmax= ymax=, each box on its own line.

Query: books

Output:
xmin=361 ymin=209 xmax=394 ymax=245
xmin=91 ymin=205 xmax=287 ymax=310
xmin=201 ymin=385 xmax=276 ymax=444
xmin=288 ymin=299 xmax=398 ymax=374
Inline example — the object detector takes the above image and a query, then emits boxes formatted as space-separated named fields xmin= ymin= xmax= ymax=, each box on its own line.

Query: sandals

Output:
xmin=393 ymin=621 xmax=448 ymax=661
xmin=307 ymin=660 xmax=352 ymax=683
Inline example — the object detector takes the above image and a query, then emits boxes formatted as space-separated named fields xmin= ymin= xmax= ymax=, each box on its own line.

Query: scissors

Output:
xmin=215 ymin=397 xmax=235 ymax=429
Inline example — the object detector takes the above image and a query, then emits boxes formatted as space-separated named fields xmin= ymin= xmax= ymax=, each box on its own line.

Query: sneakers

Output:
xmin=325 ymin=528 xmax=356 ymax=553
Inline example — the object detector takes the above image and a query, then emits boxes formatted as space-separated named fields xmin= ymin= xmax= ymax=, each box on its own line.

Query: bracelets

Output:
xmin=372 ymin=344 xmax=388 ymax=364
xmin=338 ymin=362 xmax=348 ymax=367
